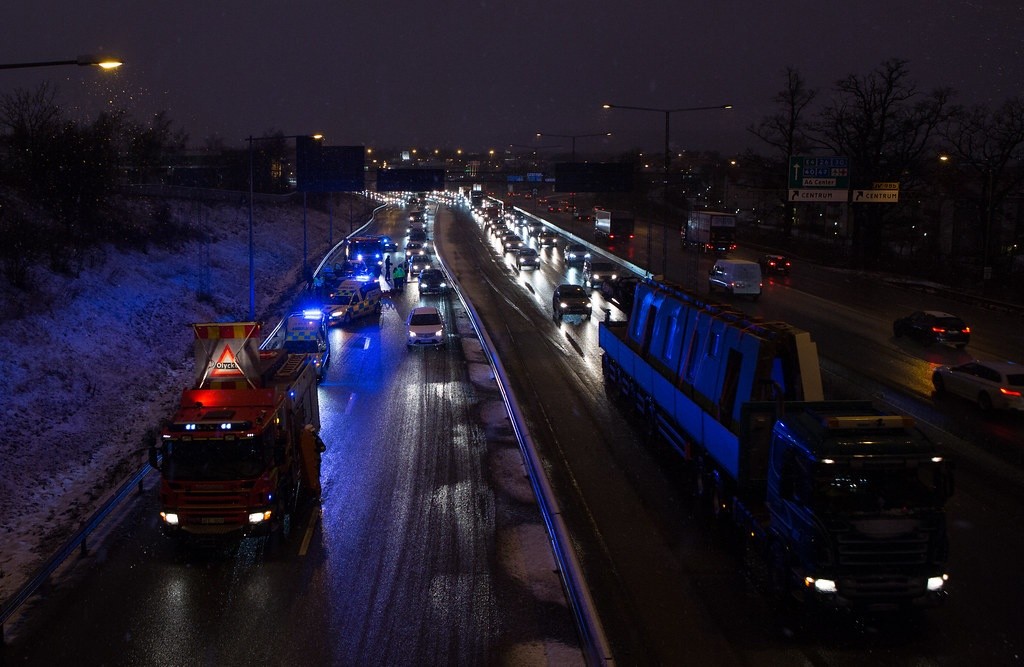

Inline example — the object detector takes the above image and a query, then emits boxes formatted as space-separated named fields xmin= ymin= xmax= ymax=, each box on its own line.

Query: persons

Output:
xmin=393 ymin=264 xmax=405 ymax=292
xmin=403 ymin=259 xmax=409 ymax=283
xmin=385 ymin=255 xmax=393 ymax=281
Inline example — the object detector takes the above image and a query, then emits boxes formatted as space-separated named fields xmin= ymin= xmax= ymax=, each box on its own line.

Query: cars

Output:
xmin=893 ymin=311 xmax=971 ymax=350
xmin=350 ymin=191 xmax=449 ymax=297
xmin=458 ymin=192 xmax=606 ymax=271
xmin=603 ymin=276 xmax=639 ymax=307
xmin=756 ymin=254 xmax=791 ymax=276
xmin=931 ymin=362 xmax=1024 ymax=421
xmin=553 ymin=284 xmax=592 ymax=318
xmin=582 ymin=257 xmax=619 ymax=288
xmin=404 ymin=306 xmax=448 ymax=352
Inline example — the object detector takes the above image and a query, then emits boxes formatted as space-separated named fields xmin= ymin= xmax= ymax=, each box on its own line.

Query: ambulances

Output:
xmin=283 ymin=314 xmax=330 ymax=381
xmin=321 ymin=280 xmax=381 ymax=327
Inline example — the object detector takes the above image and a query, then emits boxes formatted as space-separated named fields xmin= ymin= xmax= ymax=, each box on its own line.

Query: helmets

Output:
xmin=305 ymin=424 xmax=315 ymax=431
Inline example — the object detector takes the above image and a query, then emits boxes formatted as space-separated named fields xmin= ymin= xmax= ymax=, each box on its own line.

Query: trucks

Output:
xmin=593 ymin=210 xmax=635 ymax=244
xmin=680 ymin=210 xmax=737 ymax=257
xmin=147 ymin=322 xmax=327 ymax=551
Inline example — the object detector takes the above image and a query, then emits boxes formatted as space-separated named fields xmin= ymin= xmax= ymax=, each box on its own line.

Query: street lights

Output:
xmin=600 ymin=104 xmax=734 ymax=281
xmin=536 ymin=132 xmax=612 ymax=233
xmin=508 ymin=144 xmax=565 ymax=173
xmin=244 ymin=133 xmax=323 ymax=323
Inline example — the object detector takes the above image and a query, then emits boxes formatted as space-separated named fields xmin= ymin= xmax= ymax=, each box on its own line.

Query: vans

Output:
xmin=707 ymin=258 xmax=761 ymax=302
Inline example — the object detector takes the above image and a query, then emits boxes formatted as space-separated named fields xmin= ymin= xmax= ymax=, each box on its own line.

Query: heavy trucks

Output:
xmin=595 ymin=313 xmax=958 ymax=632
